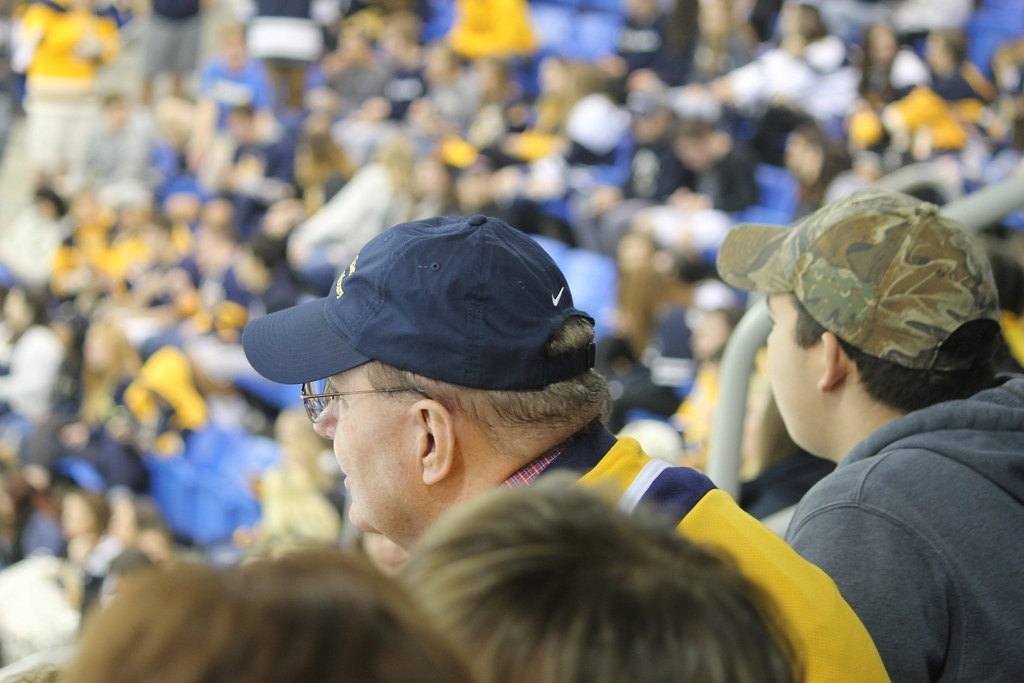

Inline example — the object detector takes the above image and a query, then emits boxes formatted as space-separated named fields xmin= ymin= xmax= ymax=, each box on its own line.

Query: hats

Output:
xmin=242 ymin=215 xmax=596 ymax=393
xmin=716 ymin=190 xmax=1001 ymax=376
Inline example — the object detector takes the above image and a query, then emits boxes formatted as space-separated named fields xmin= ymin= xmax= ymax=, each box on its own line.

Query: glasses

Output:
xmin=301 ymin=382 xmax=433 ymax=424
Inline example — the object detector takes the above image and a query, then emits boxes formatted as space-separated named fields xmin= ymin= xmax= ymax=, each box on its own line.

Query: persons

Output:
xmin=62 ymin=546 xmax=476 ymax=683
xmin=397 ymin=489 xmax=803 ymax=683
xmin=716 ymin=187 xmax=1024 ymax=683
xmin=0 ymin=0 xmax=1024 ymax=640
xmin=242 ymin=214 xmax=893 ymax=683
